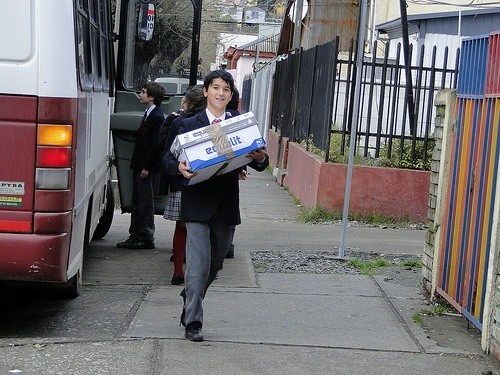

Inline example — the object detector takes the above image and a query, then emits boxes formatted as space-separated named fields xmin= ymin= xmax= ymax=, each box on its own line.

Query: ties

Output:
xmin=211 ymin=118 xmax=222 ymax=124
xmin=139 ymin=110 xmax=147 ymax=127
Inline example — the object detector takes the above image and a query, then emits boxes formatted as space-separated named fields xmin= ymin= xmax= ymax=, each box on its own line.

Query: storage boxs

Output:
xmin=171 ymin=111 xmax=267 ymax=187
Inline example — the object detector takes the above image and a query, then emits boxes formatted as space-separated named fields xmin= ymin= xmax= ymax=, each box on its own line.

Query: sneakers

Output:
xmin=170 ymin=254 xmax=174 ymax=261
xmin=179 ymin=287 xmax=203 ymax=342
xmin=116 ymin=234 xmax=156 ymax=249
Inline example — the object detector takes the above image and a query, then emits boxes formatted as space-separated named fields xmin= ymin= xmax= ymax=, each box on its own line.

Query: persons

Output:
xmin=158 ymin=84 xmax=249 ymax=285
xmin=165 ymin=70 xmax=270 ymax=341
xmin=116 ymin=82 xmax=166 ymax=249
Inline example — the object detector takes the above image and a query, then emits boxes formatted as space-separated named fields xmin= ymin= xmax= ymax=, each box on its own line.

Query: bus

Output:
xmin=0 ymin=0 xmax=203 ymax=298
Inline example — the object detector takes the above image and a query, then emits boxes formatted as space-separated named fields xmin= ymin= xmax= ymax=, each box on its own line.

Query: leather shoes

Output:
xmin=171 ymin=272 xmax=185 ymax=285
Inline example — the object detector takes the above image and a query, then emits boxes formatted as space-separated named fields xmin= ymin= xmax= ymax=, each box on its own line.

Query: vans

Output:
xmin=154 ymin=78 xmax=204 ymax=96
